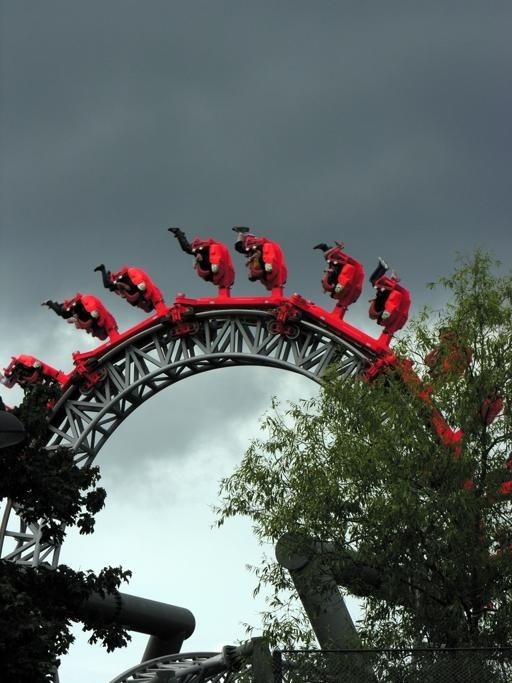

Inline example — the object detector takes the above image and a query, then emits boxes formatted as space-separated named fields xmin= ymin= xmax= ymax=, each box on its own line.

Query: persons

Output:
xmin=368 ymin=256 xmax=400 ymax=325
xmin=41 ymin=297 xmax=107 ymax=340
xmin=313 ymin=241 xmax=344 ymax=298
xmin=168 ymin=228 xmax=218 ymax=282
xmin=94 ymin=264 xmax=153 ymax=312
xmin=232 ymin=227 xmax=272 ymax=291
xmin=1 ymin=356 xmax=43 ymax=388
